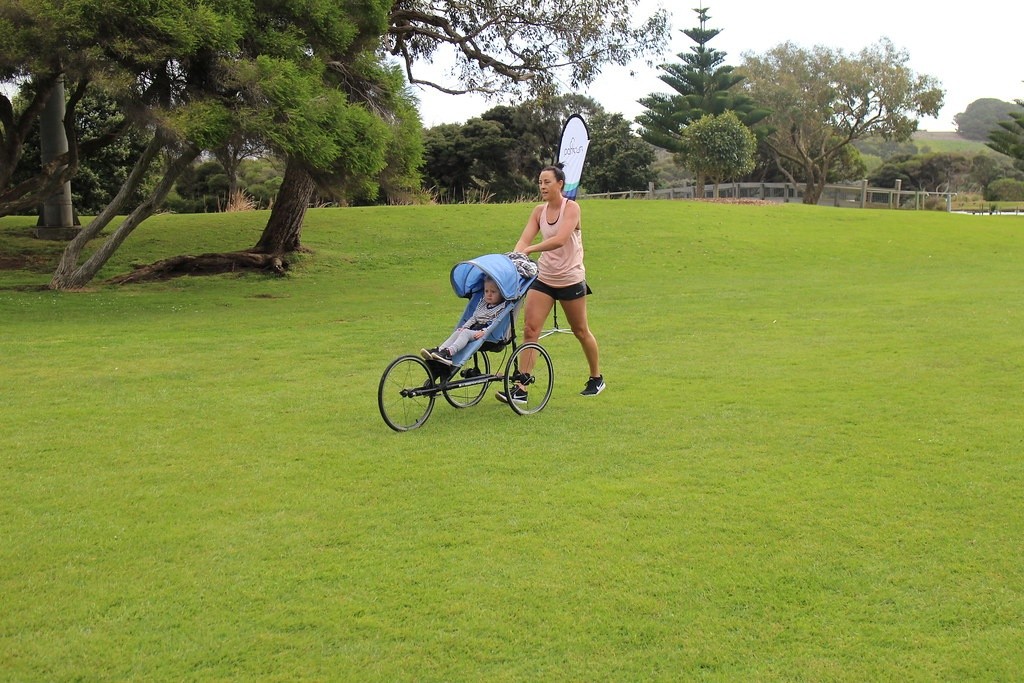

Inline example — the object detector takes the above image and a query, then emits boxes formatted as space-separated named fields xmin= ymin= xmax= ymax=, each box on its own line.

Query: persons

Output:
xmin=495 ymin=163 xmax=606 ymax=403
xmin=420 ymin=275 xmax=507 ymax=366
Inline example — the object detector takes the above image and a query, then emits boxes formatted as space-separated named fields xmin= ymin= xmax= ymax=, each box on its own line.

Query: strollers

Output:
xmin=378 ymin=251 xmax=554 ymax=432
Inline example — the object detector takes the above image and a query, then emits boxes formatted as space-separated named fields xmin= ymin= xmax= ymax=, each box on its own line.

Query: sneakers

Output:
xmin=580 ymin=375 xmax=605 ymax=397
xmin=495 ymin=385 xmax=528 ymax=404
xmin=421 ymin=348 xmax=439 ymax=359
xmin=432 ymin=350 xmax=453 ymax=364
